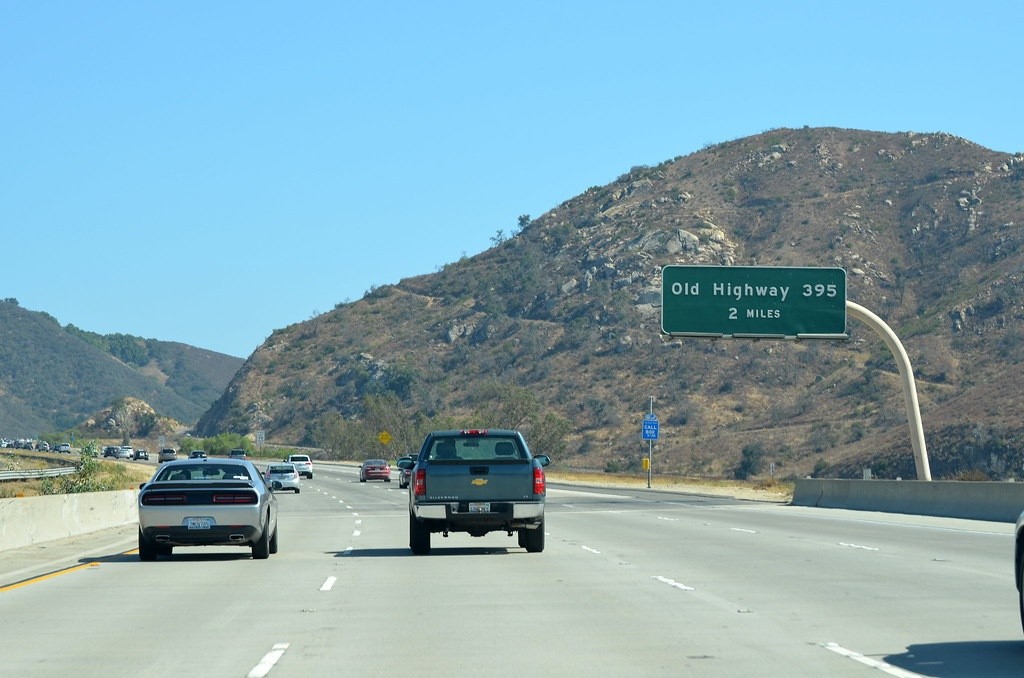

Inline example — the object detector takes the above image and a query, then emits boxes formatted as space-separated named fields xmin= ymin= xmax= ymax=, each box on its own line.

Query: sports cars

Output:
xmin=138 ymin=458 xmax=283 ymax=562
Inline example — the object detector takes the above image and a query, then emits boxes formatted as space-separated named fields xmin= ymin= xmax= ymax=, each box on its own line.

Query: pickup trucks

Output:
xmin=396 ymin=428 xmax=551 ymax=554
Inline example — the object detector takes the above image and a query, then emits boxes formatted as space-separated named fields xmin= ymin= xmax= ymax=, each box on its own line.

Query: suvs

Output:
xmin=0 ymin=437 xmax=73 ymax=454
xmin=398 ymin=452 xmax=420 ymax=489
xmin=283 ymin=454 xmax=313 ymax=479
xmin=226 ymin=448 xmax=247 ymax=460
xmin=157 ymin=447 xmax=178 ymax=463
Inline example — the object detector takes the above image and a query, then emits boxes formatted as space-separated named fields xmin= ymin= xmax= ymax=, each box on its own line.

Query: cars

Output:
xmin=1015 ymin=509 xmax=1024 ymax=633
xmin=186 ymin=450 xmax=209 ymax=460
xmin=358 ymin=459 xmax=391 ymax=483
xmin=132 ymin=449 xmax=150 ymax=461
xmin=100 ymin=445 xmax=135 ymax=460
xmin=260 ymin=462 xmax=300 ymax=494
